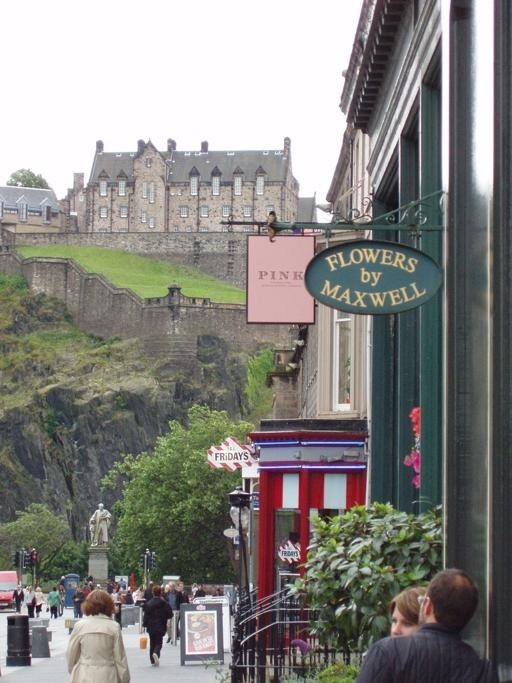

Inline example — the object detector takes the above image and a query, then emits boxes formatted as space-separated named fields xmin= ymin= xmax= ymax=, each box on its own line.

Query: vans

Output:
xmin=0 ymin=570 xmax=21 ymax=608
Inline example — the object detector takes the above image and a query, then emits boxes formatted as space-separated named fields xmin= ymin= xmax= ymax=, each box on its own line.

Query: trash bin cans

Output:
xmin=6 ymin=615 xmax=31 ymax=666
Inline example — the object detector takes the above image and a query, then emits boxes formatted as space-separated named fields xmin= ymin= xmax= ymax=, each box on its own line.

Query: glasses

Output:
xmin=418 ymin=596 xmax=428 ymax=606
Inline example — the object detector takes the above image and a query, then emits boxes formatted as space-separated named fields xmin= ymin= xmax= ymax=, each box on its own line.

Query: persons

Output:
xmin=353 ymin=569 xmax=483 ymax=682
xmin=388 ymin=585 xmax=429 ymax=636
xmin=63 ymin=590 xmax=130 ymax=683
xmin=87 ymin=503 xmax=114 ymax=546
xmin=10 ymin=573 xmax=247 ymax=667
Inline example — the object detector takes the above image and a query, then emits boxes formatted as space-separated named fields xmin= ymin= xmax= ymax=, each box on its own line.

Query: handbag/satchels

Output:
xmin=140 ymin=635 xmax=147 ymax=650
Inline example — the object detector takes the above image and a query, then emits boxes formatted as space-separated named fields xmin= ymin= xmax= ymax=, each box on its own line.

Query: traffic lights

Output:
xmin=31 ymin=550 xmax=38 ymax=561
xmin=148 ymin=552 xmax=157 ymax=569
xmin=140 ymin=554 xmax=145 ymax=568
xmin=23 ymin=551 xmax=30 ymax=568
xmin=12 ymin=551 xmax=19 ymax=567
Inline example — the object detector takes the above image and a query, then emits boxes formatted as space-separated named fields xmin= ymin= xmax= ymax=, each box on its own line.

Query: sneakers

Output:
xmin=151 ymin=652 xmax=160 ymax=667
